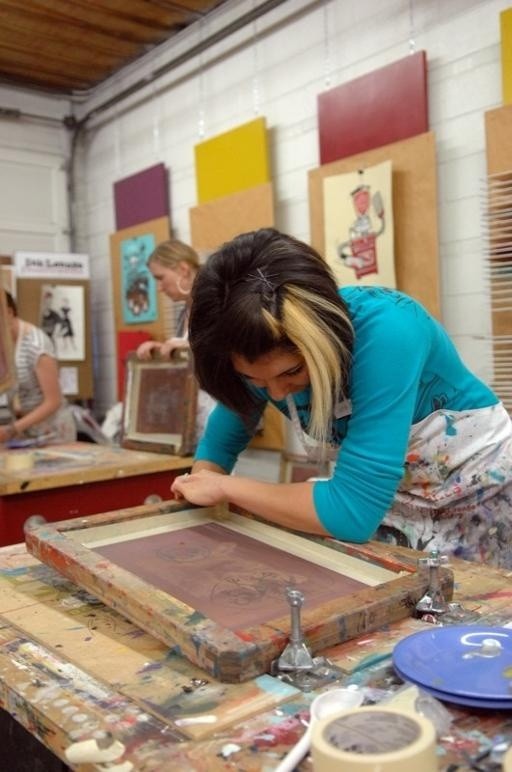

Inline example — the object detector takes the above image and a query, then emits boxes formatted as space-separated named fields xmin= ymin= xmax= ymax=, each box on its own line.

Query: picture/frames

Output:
xmin=119 ymin=350 xmax=199 ymax=456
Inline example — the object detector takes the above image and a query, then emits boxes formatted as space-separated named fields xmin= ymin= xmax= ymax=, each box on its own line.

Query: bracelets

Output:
xmin=8 ymin=423 xmax=19 ymax=437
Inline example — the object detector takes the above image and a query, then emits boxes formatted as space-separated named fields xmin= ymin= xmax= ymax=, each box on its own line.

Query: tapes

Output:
xmin=310 ymin=685 xmax=440 ymax=772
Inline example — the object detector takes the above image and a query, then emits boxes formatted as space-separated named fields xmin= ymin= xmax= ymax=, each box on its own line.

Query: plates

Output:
xmin=391 ymin=623 xmax=512 ymax=712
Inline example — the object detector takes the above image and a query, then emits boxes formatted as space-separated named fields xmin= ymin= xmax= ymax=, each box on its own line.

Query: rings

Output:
xmin=184 ymin=473 xmax=189 ymax=480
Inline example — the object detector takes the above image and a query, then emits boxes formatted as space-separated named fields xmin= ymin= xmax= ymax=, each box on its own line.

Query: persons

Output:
xmin=137 ymin=238 xmax=201 ymax=360
xmin=0 ymin=285 xmax=83 ymax=447
xmin=168 ymin=225 xmax=512 ymax=574
xmin=41 ymin=290 xmax=76 ymax=353
